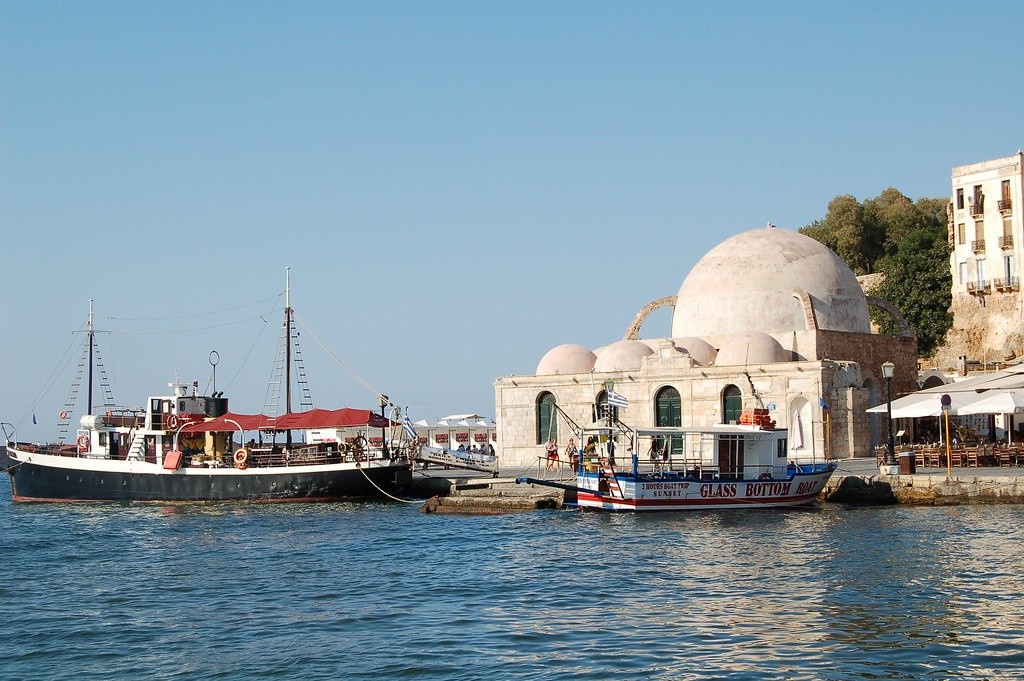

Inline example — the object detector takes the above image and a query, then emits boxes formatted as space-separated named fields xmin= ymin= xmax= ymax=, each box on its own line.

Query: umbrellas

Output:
xmin=791 ymin=410 xmax=804 ymax=474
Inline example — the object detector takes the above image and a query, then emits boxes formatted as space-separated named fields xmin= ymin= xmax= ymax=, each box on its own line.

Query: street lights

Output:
xmin=881 ymin=360 xmax=900 ymax=467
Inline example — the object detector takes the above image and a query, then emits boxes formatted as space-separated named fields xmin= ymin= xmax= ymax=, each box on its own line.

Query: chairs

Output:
xmin=876 ymin=448 xmax=887 ymax=467
xmin=913 ymin=445 xmax=923 ymax=451
xmin=928 ymin=450 xmax=945 ymax=468
xmin=950 ymin=450 xmax=966 ymax=467
xmin=999 ymin=449 xmax=1018 ymax=467
xmin=915 ymin=449 xmax=929 ymax=467
xmin=966 ymin=449 xmax=983 ymax=467
xmin=992 ymin=443 xmax=1006 ymax=465
xmin=895 ymin=445 xmax=910 ymax=463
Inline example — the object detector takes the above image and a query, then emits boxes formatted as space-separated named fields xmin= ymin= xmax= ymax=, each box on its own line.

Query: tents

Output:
xmin=865 ymin=363 xmax=1024 ymax=445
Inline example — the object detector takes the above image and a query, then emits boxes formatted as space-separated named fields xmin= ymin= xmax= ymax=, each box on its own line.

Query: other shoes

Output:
xmin=551 ymin=468 xmax=555 ymax=472
xmin=557 ymin=469 xmax=561 ymax=472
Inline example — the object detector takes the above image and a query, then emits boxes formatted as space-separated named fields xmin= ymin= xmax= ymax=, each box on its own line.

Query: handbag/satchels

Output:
xmin=548 ymin=444 xmax=553 ymax=456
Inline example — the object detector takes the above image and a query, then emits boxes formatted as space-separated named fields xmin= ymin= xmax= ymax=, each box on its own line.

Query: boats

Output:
xmin=1 ymin=265 xmax=415 ymax=505
xmin=515 ymin=392 xmax=841 ymax=513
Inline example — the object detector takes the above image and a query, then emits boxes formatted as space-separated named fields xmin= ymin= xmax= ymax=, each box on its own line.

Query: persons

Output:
xmin=247 ymin=439 xmax=258 ymax=450
xmin=545 ymin=433 xmax=673 ymax=474
xmin=919 ymin=429 xmax=1023 ymax=446
xmin=457 ymin=444 xmax=495 ymax=456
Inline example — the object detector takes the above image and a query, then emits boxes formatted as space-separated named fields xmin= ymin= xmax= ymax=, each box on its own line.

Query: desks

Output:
xmin=876 ymin=443 xmax=1024 ymax=466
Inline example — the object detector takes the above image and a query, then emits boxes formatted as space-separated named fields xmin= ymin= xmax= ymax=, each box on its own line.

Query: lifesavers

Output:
xmin=59 ymin=410 xmax=67 ymax=419
xmin=233 ymin=448 xmax=248 ymax=463
xmin=106 ymin=411 xmax=113 ymax=416
xmin=77 ymin=435 xmax=89 ymax=448
xmin=758 ymin=473 xmax=771 ymax=480
xmin=235 ymin=461 xmax=248 ymax=469
xmin=337 ymin=442 xmax=346 ymax=452
xmin=167 ymin=415 xmax=179 ymax=429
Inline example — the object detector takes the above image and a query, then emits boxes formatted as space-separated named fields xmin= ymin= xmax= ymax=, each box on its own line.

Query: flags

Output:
xmin=607 ymin=390 xmax=629 ymax=408
xmin=402 ymin=417 xmax=416 ymax=440
xmin=32 ymin=415 xmax=36 ymax=424
